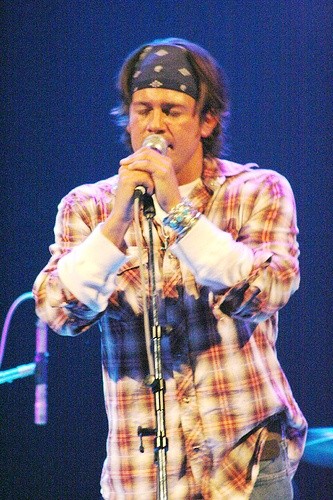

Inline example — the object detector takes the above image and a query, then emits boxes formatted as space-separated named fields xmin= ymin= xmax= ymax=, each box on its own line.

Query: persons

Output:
xmin=32 ymin=36 xmax=308 ymax=500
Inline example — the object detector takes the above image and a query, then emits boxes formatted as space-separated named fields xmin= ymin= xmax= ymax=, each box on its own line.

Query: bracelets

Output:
xmin=159 ymin=198 xmax=202 ymax=251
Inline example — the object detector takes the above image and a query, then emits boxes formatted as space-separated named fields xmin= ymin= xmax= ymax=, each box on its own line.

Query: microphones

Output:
xmin=133 ymin=135 xmax=167 ymax=201
xmin=34 ymin=320 xmax=48 ymax=424
